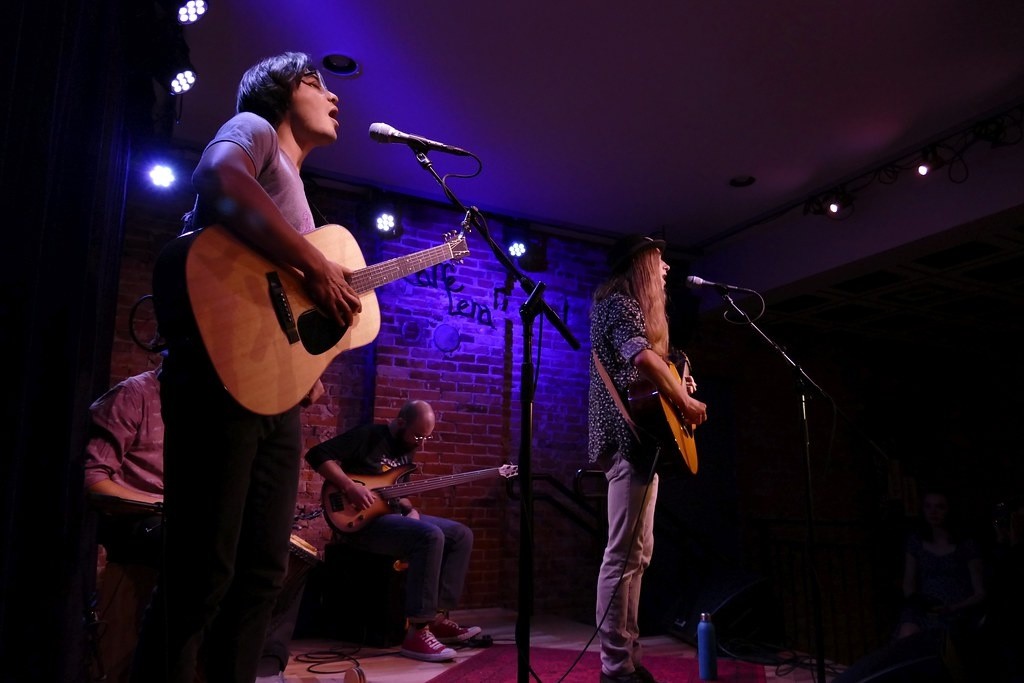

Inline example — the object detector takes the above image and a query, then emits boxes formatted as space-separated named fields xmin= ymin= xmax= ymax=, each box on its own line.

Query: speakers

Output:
xmin=831 ymin=625 xmax=964 ymax=683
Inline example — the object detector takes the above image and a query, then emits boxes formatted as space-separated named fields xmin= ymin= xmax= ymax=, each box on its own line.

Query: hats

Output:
xmin=610 ymin=232 xmax=666 ymax=279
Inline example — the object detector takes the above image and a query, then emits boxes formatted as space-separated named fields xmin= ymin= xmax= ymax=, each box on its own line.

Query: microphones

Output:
xmin=687 ymin=276 xmax=757 ymax=294
xmin=368 ymin=122 xmax=472 ymax=157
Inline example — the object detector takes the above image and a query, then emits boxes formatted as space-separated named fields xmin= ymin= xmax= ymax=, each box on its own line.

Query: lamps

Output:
xmin=138 ymin=23 xmax=198 ymax=96
xmin=501 ymin=221 xmax=527 ymax=258
xmin=143 ymin=142 xmax=187 ymax=194
xmin=918 ymin=145 xmax=946 ymax=177
xmin=365 ymin=189 xmax=404 ymax=241
xmin=151 ymin=0 xmax=210 ymax=25
xmin=830 ymin=188 xmax=857 ymax=213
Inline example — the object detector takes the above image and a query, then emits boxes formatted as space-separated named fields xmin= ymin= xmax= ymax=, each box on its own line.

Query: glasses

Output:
xmin=305 ymin=69 xmax=330 ymax=95
xmin=410 ymin=432 xmax=434 ymax=442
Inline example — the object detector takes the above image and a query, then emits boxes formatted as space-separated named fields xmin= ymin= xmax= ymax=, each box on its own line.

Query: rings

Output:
xmin=698 ymin=414 xmax=702 ymax=417
xmin=336 ymin=294 xmax=342 ymax=301
xmin=361 ymin=503 xmax=364 ymax=508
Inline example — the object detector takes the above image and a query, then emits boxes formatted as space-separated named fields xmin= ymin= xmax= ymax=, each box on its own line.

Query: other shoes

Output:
xmin=600 ymin=665 xmax=658 ymax=683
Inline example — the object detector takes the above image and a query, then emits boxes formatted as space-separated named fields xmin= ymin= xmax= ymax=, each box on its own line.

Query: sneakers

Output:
xmin=431 ymin=612 xmax=481 ymax=643
xmin=400 ymin=626 xmax=458 ymax=661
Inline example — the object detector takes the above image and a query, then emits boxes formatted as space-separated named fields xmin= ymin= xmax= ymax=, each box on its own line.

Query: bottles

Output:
xmin=697 ymin=612 xmax=717 ymax=679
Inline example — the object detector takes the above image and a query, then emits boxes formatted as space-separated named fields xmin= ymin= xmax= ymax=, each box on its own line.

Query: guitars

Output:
xmin=628 ymin=351 xmax=700 ymax=491
xmin=319 ymin=460 xmax=520 ymax=536
xmin=152 ymin=222 xmax=472 ymax=436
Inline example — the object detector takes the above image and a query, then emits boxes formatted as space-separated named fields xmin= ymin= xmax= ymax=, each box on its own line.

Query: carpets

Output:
xmin=424 ymin=644 xmax=768 ymax=683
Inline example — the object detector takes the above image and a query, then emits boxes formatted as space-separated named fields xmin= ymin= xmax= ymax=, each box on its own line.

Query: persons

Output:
xmin=82 ymin=352 xmax=306 ymax=683
xmin=305 ymin=400 xmax=481 ymax=661
xmin=896 ymin=492 xmax=1024 ymax=639
xmin=157 ymin=50 xmax=362 ymax=683
xmin=586 ymin=235 xmax=707 ymax=683
